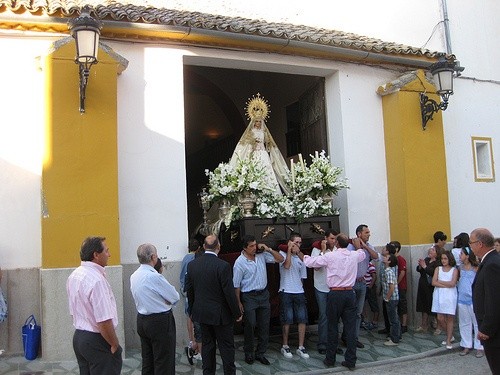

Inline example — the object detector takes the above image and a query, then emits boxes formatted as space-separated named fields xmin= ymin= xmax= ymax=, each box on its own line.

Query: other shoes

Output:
xmin=318 ymin=348 xmax=325 ymax=353
xmin=446 ymin=345 xmax=452 ymax=349
xmin=341 ymin=361 xmax=354 ymax=370
xmin=434 ymin=329 xmax=441 ymax=335
xmin=401 ymin=327 xmax=408 ymax=333
xmin=185 ymin=347 xmax=194 ymax=366
xmin=336 ymin=348 xmax=343 ymax=353
xmin=459 ymin=351 xmax=466 ymax=355
xmin=324 ymin=360 xmax=333 ymax=368
xmin=476 ymin=353 xmax=482 ymax=357
xmin=377 ymin=328 xmax=389 ymax=334
xmin=360 ymin=321 xmax=369 ymax=327
xmin=443 ymin=337 xmax=455 ymax=345
xmin=356 ymin=342 xmax=364 ymax=348
xmin=365 ymin=321 xmax=377 ymax=329
xmin=415 ymin=326 xmax=424 ymax=331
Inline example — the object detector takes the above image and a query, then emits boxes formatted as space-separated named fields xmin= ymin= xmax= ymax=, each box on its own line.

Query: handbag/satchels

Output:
xmin=427 ymin=274 xmax=432 ymax=284
xmin=22 ymin=314 xmax=41 ymax=360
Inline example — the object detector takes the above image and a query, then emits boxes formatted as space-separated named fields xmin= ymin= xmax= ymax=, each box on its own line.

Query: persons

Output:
xmin=129 ymin=225 xmax=500 ymax=375
xmin=67 ymin=236 xmax=124 ymax=375
xmin=240 ymin=116 xmax=282 ymax=200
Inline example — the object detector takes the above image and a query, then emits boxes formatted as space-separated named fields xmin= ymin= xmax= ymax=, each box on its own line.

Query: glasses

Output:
xmin=468 ymin=241 xmax=484 ymax=245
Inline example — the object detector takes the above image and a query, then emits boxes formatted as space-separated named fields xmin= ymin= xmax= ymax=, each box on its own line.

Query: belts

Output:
xmin=356 ymin=276 xmax=364 ymax=282
xmin=330 ymin=287 xmax=354 ymax=290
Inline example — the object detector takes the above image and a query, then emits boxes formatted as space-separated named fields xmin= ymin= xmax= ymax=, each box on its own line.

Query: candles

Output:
xmin=290 ymin=159 xmax=295 ymax=188
xmin=298 ymin=153 xmax=303 ymax=166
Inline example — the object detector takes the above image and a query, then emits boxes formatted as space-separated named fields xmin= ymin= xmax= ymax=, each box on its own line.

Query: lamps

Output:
xmin=199 ymin=188 xmax=211 ymax=232
xmin=420 ymin=55 xmax=456 ymax=129
xmin=70 ymin=6 xmax=104 ymax=115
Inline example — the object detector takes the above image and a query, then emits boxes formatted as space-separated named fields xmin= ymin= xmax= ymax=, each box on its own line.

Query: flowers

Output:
xmin=205 ymin=156 xmax=278 ymax=197
xmin=286 ymin=150 xmax=351 ymax=197
xmin=254 ymin=193 xmax=340 ymax=217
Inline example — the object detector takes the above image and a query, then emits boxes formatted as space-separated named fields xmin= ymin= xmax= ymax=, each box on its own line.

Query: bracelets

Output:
xmin=384 ymin=298 xmax=389 ymax=302
xmin=297 ymin=250 xmax=300 ymax=254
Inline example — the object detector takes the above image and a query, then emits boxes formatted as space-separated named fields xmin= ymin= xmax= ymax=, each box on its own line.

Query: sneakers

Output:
xmin=296 ymin=346 xmax=309 ymax=359
xmin=280 ymin=344 xmax=292 ymax=359
xmin=384 ymin=340 xmax=398 ymax=346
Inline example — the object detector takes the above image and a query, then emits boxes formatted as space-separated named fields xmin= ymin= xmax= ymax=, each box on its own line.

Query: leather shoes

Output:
xmin=245 ymin=354 xmax=254 ymax=364
xmin=256 ymin=355 xmax=270 ymax=365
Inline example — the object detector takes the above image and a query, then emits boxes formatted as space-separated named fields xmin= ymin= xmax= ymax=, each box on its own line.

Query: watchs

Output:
xmin=320 ymin=251 xmax=324 ymax=255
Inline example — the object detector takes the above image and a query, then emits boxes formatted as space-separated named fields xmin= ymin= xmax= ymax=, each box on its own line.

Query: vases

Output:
xmin=239 ymin=191 xmax=257 ymax=218
